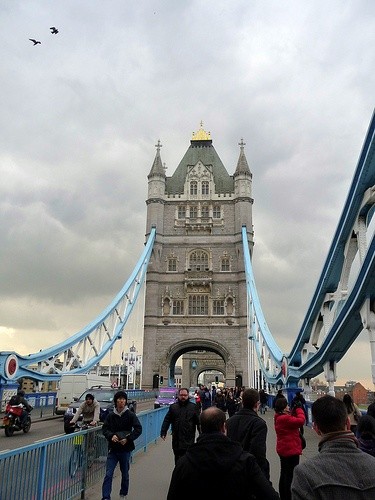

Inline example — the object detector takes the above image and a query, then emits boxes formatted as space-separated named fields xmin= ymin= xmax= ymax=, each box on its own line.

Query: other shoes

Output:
xmin=119 ymin=491 xmax=128 ymax=500
xmin=263 ymin=411 xmax=265 ymax=414
xmin=261 ymin=412 xmax=262 ymax=414
xmin=102 ymin=499 xmax=110 ymax=500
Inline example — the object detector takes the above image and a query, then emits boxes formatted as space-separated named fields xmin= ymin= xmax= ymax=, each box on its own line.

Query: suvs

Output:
xmin=63 ymin=385 xmax=137 ymax=435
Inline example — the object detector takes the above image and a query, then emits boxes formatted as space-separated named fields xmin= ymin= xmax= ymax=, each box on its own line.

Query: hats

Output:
xmin=216 ymin=389 xmax=221 ymax=394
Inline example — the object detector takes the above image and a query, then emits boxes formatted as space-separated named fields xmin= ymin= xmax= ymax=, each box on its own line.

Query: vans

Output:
xmin=55 ymin=373 xmax=113 ymax=416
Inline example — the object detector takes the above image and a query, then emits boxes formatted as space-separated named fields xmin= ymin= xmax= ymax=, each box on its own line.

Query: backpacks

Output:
xmin=9 ymin=396 xmax=20 ymax=405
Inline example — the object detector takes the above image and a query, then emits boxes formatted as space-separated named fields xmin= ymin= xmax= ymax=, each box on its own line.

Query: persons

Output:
xmin=226 ymin=389 xmax=272 ymax=486
xmin=356 ymin=415 xmax=375 ymax=457
xmin=343 ymin=394 xmax=362 ymax=434
xmin=112 ymin=381 xmax=118 ymax=388
xmin=167 ymin=407 xmax=280 ymax=500
xmin=291 ymin=394 xmax=375 ymax=500
xmin=272 ymin=390 xmax=285 ymax=409
xmin=101 ymin=391 xmax=142 ymax=500
xmin=290 ymin=391 xmax=306 ymax=409
xmin=69 ymin=394 xmax=100 ymax=460
xmin=160 ymin=388 xmax=201 ymax=465
xmin=6 ymin=390 xmax=33 ymax=430
xmin=194 ymin=385 xmax=268 ymax=417
xmin=272 ymin=398 xmax=305 ymax=500
xmin=367 ymin=392 xmax=375 ymax=417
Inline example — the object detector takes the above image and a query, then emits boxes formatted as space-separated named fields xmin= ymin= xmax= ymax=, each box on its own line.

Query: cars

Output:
xmin=189 ymin=387 xmax=200 ymax=398
xmin=153 ymin=388 xmax=177 ymax=409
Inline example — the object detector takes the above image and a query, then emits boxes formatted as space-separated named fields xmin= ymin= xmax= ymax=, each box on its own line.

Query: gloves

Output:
xmin=294 ymin=399 xmax=303 ymax=409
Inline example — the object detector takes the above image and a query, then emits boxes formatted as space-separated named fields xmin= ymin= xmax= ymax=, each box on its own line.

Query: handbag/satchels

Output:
xmin=352 ymin=403 xmax=359 ymax=422
xmin=299 ymin=432 xmax=307 ymax=451
xmin=5 ymin=405 xmax=23 ymax=416
xmin=196 ymin=397 xmax=201 ymax=403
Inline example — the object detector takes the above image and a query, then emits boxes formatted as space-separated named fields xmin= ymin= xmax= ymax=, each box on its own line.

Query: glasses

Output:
xmin=179 ymin=394 xmax=189 ymax=396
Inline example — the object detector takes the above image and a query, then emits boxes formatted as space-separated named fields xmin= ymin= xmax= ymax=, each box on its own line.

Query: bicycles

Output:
xmin=68 ymin=423 xmax=98 ymax=478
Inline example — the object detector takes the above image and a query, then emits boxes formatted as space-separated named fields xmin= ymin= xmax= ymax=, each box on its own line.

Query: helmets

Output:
xmin=17 ymin=390 xmax=25 ymax=396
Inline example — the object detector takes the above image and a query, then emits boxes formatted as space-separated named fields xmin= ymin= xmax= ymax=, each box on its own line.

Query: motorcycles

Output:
xmin=2 ymin=404 xmax=32 ymax=437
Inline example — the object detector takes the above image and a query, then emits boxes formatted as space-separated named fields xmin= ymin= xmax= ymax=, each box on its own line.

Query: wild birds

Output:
xmin=29 ymin=39 xmax=41 ymax=46
xmin=50 ymin=27 xmax=59 ymax=34
